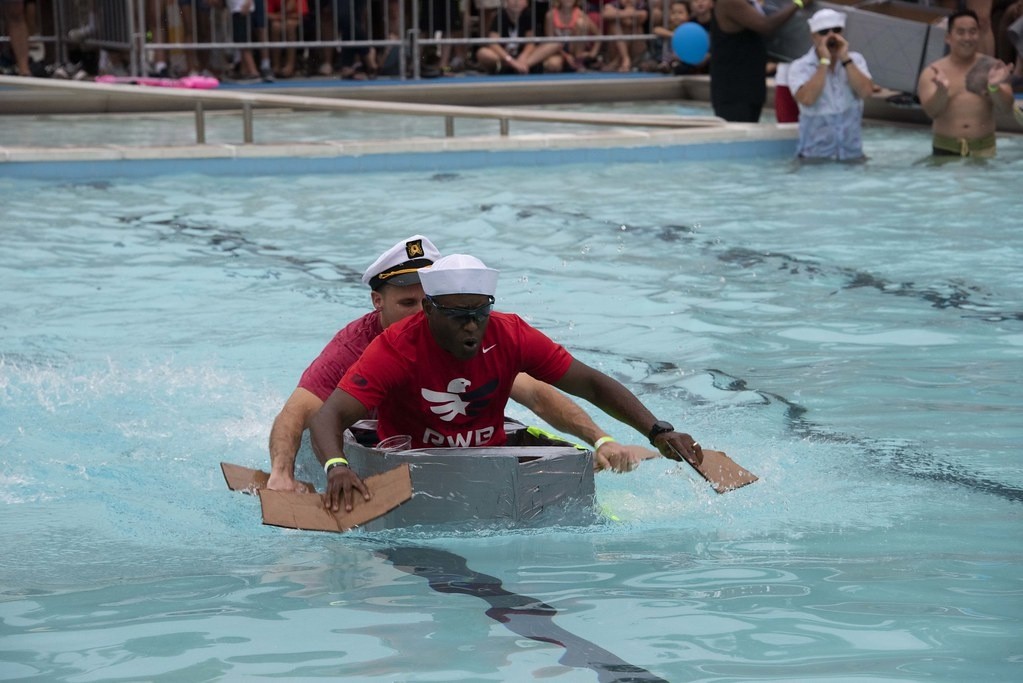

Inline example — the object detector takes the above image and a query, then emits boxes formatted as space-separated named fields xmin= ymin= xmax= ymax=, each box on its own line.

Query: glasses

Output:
xmin=426 ymin=295 xmax=495 ymax=321
xmin=819 ymin=28 xmax=841 ymax=35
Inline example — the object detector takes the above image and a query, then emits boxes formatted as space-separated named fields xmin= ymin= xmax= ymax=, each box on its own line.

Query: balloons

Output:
xmin=671 ymin=21 xmax=710 ymax=65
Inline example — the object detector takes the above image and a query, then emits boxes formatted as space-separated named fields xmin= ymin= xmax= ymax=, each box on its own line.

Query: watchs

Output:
xmin=648 ymin=420 xmax=674 ymax=447
xmin=502 ymin=55 xmax=513 ymax=63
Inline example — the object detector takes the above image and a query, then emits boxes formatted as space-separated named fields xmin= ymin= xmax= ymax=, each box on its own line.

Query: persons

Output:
xmin=710 ymin=0 xmax=1023 ymax=163
xmin=310 ymin=254 xmax=704 ymax=512
xmin=0 ymin=0 xmax=713 ymax=85
xmin=267 ymin=235 xmax=639 ymax=493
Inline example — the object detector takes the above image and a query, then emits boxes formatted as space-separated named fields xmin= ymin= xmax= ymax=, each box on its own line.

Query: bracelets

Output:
xmin=842 ymin=59 xmax=852 ymax=66
xmin=324 ymin=457 xmax=351 ymax=476
xmin=820 ymin=57 xmax=831 ymax=64
xmin=988 ymin=85 xmax=1000 ymax=93
xmin=594 ymin=436 xmax=616 ymax=449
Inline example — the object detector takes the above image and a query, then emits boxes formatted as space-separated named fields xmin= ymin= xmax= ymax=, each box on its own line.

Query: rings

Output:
xmin=692 ymin=442 xmax=698 ymax=446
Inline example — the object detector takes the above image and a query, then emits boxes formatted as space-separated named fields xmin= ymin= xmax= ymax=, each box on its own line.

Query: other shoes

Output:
xmin=262 ymin=68 xmax=273 ymax=81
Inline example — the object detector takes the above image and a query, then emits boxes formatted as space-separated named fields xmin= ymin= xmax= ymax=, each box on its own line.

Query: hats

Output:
xmin=417 ymin=255 xmax=500 ymax=296
xmin=808 ymin=9 xmax=846 ymax=33
xmin=362 ymin=235 xmax=441 ymax=287
